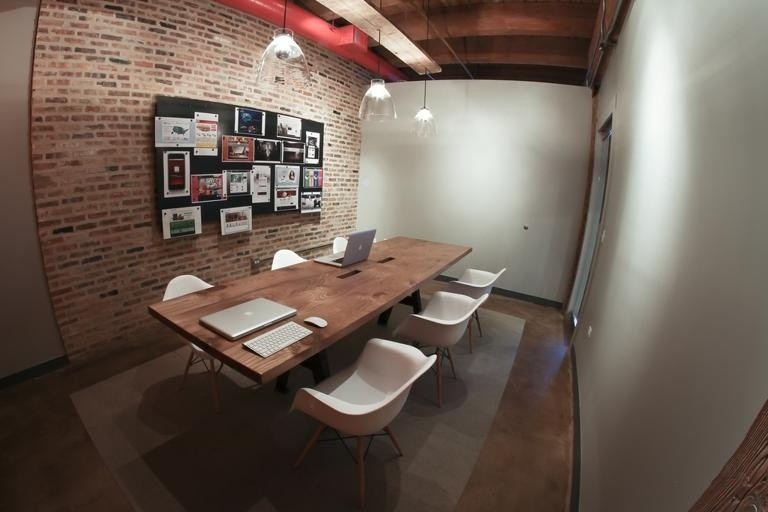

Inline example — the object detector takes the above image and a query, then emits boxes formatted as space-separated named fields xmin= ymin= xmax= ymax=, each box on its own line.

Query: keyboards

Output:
xmin=243 ymin=321 xmax=313 ymax=358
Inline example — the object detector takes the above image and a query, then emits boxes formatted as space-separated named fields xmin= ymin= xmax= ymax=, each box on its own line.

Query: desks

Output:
xmin=147 ymin=236 xmax=473 ymax=393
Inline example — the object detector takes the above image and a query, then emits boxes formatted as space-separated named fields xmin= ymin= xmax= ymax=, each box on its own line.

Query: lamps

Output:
xmin=256 ymin=0 xmax=312 ymax=91
xmin=412 ymin=0 xmax=436 ymax=138
xmin=358 ymin=0 xmax=397 ymax=123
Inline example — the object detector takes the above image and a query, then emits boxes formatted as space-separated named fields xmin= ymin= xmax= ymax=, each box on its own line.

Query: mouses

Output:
xmin=304 ymin=316 xmax=328 ymax=328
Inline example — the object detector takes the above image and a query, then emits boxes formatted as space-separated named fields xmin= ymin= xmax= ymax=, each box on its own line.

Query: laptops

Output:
xmin=313 ymin=229 xmax=376 ymax=268
xmin=199 ymin=297 xmax=297 ymax=340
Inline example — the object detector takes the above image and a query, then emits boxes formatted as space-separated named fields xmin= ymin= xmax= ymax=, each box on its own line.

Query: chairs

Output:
xmin=447 ymin=267 xmax=508 ymax=354
xmin=288 ymin=337 xmax=438 ymax=512
xmin=391 ymin=291 xmax=489 ymax=408
xmin=333 ymin=237 xmax=348 ymax=254
xmin=162 ymin=275 xmax=224 ymax=414
xmin=271 ymin=249 xmax=309 ymax=271
